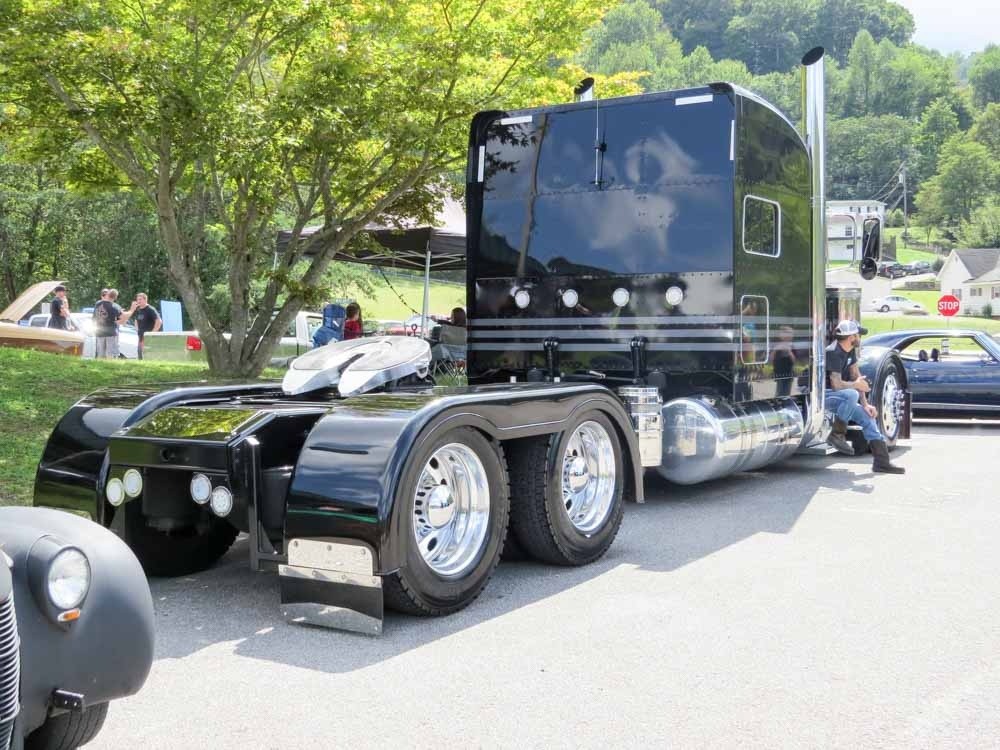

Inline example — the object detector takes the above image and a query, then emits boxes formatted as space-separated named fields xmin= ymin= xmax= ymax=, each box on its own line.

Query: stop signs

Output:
xmin=937 ymin=295 xmax=960 ymax=317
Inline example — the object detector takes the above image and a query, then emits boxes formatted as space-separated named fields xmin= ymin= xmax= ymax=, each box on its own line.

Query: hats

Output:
xmin=56 ymin=286 xmax=69 ymax=291
xmin=835 ymin=319 xmax=868 ymax=335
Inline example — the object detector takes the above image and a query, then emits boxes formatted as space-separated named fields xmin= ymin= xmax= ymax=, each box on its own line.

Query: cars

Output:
xmin=903 ymin=260 xmax=931 ymax=275
xmin=859 ymin=330 xmax=1000 ymax=423
xmin=0 ymin=280 xmax=456 ymax=364
xmin=870 ymin=295 xmax=921 ymax=313
xmin=0 ymin=503 xmax=156 ymax=749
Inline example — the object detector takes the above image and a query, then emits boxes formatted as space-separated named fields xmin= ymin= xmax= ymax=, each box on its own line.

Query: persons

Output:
xmin=772 ymin=326 xmax=797 ymax=396
xmin=863 ymin=230 xmax=869 ymax=247
xmin=429 ymin=308 xmax=467 ymax=375
xmin=825 ymin=320 xmax=905 ymax=474
xmin=735 ymin=300 xmax=758 ymax=403
xmin=52 ymin=285 xmax=71 ymax=329
xmin=92 ymin=288 xmax=141 ymax=361
xmin=344 ymin=303 xmax=364 ymax=339
xmin=134 ymin=293 xmax=162 ymax=361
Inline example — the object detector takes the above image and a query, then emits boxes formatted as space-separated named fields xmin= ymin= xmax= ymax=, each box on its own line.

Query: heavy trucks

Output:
xmin=34 ymin=44 xmax=913 ymax=639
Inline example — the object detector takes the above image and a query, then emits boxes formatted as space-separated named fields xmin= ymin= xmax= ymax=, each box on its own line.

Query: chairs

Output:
xmin=431 ymin=344 xmax=467 ymax=386
xmin=918 ymin=349 xmax=940 ymax=362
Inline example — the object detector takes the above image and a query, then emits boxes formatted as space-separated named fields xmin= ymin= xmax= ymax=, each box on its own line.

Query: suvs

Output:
xmin=879 ymin=262 xmax=907 ymax=279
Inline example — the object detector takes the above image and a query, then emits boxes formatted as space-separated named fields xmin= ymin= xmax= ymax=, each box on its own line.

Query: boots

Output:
xmin=869 ymin=447 xmax=905 ymax=474
xmin=827 ymin=420 xmax=855 ymax=456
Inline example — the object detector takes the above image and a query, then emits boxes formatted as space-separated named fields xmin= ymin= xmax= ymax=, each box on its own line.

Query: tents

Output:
xmin=273 ymin=197 xmax=469 ymax=344
